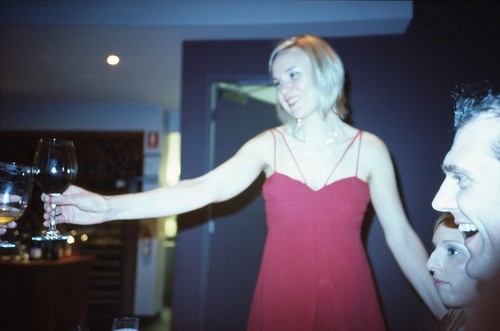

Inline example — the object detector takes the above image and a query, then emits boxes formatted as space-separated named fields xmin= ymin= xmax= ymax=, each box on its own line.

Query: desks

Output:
xmin=1 ymin=252 xmax=95 ymax=330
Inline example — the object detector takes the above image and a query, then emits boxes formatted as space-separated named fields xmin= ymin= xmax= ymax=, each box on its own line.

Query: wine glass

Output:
xmin=31 ymin=137 xmax=79 ymax=241
xmin=0 ymin=162 xmax=31 ymax=248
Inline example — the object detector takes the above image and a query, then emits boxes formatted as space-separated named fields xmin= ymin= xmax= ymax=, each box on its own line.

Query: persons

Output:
xmin=426 ymin=212 xmax=491 ymax=331
xmin=43 ymin=34 xmax=448 ymax=331
xmin=429 ymin=82 xmax=500 ymax=281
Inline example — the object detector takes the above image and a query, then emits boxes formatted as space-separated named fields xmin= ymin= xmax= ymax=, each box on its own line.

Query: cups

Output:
xmin=112 ymin=317 xmax=139 ymax=331
